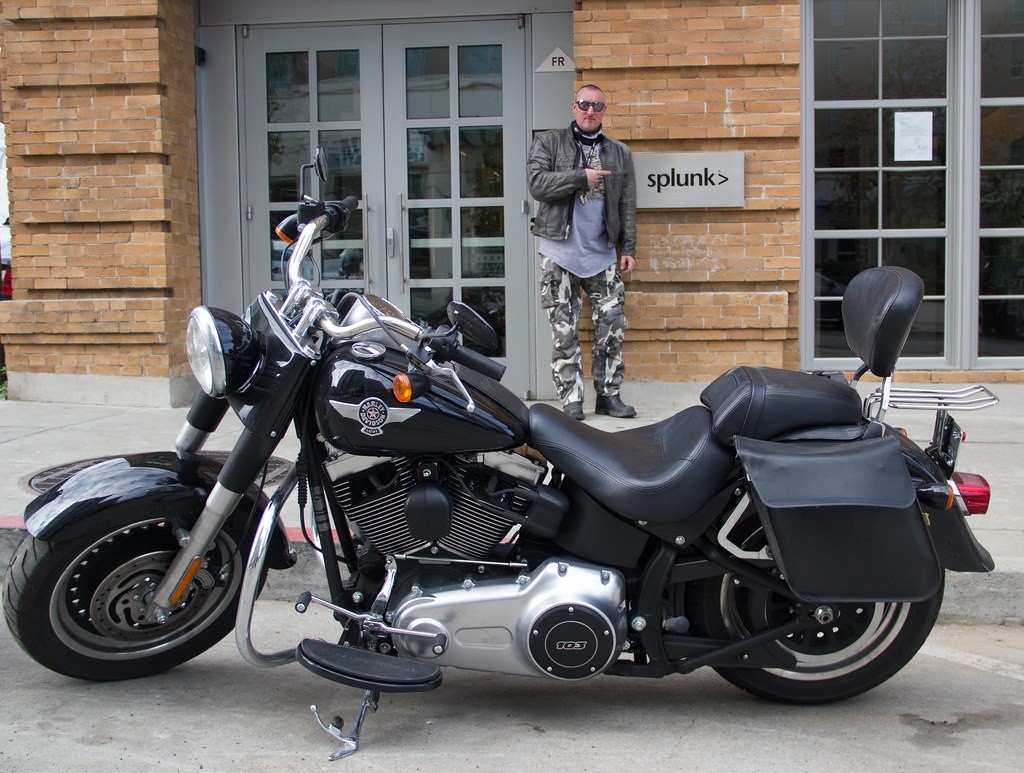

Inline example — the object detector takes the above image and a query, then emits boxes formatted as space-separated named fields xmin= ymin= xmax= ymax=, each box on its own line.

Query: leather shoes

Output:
xmin=563 ymin=400 xmax=586 ymax=421
xmin=595 ymin=396 xmax=637 ymax=418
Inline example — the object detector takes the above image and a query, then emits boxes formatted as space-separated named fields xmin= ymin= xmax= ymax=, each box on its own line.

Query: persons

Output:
xmin=524 ymin=84 xmax=638 ymax=420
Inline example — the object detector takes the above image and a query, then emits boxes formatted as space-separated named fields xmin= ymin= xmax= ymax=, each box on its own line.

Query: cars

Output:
xmin=813 ymin=272 xmax=849 ymax=327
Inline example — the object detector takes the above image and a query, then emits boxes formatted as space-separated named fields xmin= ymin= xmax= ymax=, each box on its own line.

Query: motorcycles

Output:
xmin=2 ymin=149 xmax=998 ymax=706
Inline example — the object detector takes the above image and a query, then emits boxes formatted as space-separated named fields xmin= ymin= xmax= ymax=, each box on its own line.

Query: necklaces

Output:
xmin=577 ymin=137 xmax=596 ymax=169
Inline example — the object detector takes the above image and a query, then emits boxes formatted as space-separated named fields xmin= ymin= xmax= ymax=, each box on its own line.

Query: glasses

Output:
xmin=575 ymin=100 xmax=605 ymax=112
